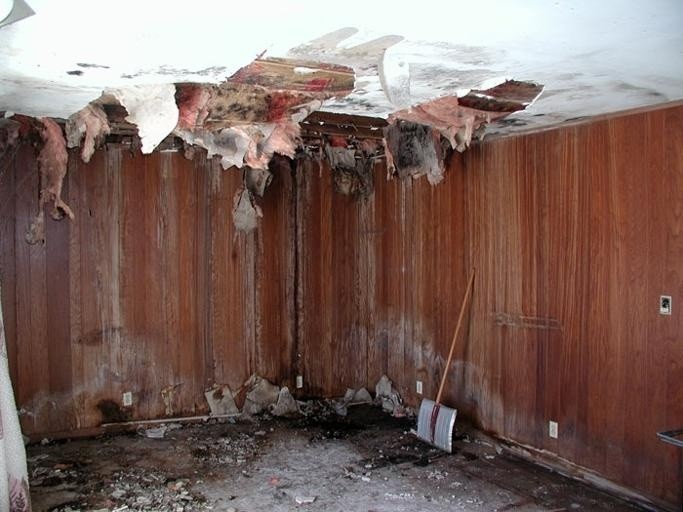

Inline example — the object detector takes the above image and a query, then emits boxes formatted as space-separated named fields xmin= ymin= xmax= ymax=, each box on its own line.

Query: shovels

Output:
xmin=417 ymin=269 xmax=474 ymax=453
